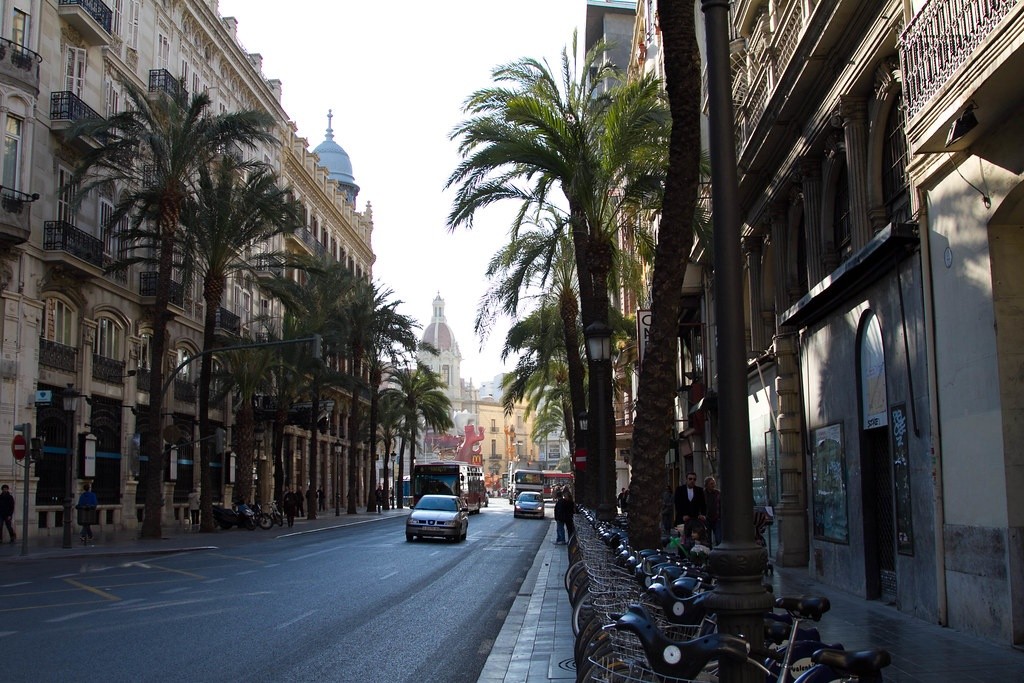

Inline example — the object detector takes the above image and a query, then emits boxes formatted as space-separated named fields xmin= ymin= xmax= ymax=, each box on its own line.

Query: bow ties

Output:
xmin=688 ymin=486 xmax=693 ymax=489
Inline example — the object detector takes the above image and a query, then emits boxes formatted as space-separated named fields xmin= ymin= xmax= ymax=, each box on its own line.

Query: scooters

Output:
xmin=213 ymin=497 xmax=273 ymax=530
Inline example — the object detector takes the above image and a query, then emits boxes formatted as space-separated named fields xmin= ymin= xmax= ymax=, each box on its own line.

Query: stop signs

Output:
xmin=575 ymin=449 xmax=587 ymax=471
xmin=11 ymin=436 xmax=27 ymax=460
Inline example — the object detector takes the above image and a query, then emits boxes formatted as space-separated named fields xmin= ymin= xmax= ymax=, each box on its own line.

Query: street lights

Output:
xmin=14 ymin=424 xmax=31 ymax=555
xmin=334 ymin=439 xmax=342 ymax=516
xmin=586 ymin=318 xmax=615 ymax=503
xmin=390 ymin=451 xmax=396 ymax=509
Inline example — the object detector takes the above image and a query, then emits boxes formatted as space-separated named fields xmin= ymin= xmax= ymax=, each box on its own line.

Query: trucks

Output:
xmin=506 ymin=460 xmax=544 ymax=504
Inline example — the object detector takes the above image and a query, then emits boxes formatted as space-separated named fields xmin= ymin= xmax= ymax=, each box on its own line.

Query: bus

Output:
xmin=394 ymin=475 xmax=411 ymax=505
xmin=409 ymin=460 xmax=487 ymax=515
xmin=500 ymin=470 xmax=573 ymax=500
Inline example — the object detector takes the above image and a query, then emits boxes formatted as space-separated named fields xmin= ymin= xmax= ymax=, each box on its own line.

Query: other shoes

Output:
xmin=88 ymin=536 xmax=94 ymax=540
xmin=0 ymin=540 xmax=3 ymax=544
xmin=553 ymin=541 xmax=565 ymax=545
xmin=80 ymin=537 xmax=85 ymax=541
xmin=10 ymin=536 xmax=15 ymax=544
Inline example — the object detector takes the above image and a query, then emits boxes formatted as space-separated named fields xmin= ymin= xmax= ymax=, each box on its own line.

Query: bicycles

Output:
xmin=564 ymin=503 xmax=891 ymax=683
xmin=266 ymin=500 xmax=283 ymax=528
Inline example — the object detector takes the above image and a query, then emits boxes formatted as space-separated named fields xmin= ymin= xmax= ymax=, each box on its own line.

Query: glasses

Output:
xmin=687 ymin=477 xmax=697 ymax=481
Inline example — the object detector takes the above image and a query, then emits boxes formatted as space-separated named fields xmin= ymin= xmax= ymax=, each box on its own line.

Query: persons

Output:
xmin=375 ymin=486 xmax=383 ymax=513
xmin=389 ymin=486 xmax=394 ymax=505
xmin=689 ymin=525 xmax=708 ymax=549
xmin=188 ymin=489 xmax=200 ymax=525
xmin=551 ymin=484 xmax=574 ymax=546
xmin=702 ymin=476 xmax=721 ymax=547
xmin=0 ymin=485 xmax=17 ymax=543
xmin=306 ymin=485 xmax=318 ymax=512
xmin=78 ymin=484 xmax=97 ymax=541
xmin=617 ymin=487 xmax=630 ymax=514
xmin=317 ymin=486 xmax=325 ymax=512
xmin=285 ymin=486 xmax=295 ymax=527
xmin=294 ymin=485 xmax=305 ymax=517
xmin=674 ymin=474 xmax=707 ymax=538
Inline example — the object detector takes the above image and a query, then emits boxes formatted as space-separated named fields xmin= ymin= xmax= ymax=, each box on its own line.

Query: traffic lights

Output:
xmin=313 ymin=334 xmax=324 ymax=360
xmin=217 ymin=428 xmax=227 ymax=454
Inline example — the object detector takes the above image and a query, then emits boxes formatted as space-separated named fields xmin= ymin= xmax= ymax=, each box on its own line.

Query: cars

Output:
xmin=405 ymin=494 xmax=469 ymax=543
xmin=752 ymin=476 xmax=767 ymax=495
xmin=514 ymin=491 xmax=547 ymax=518
